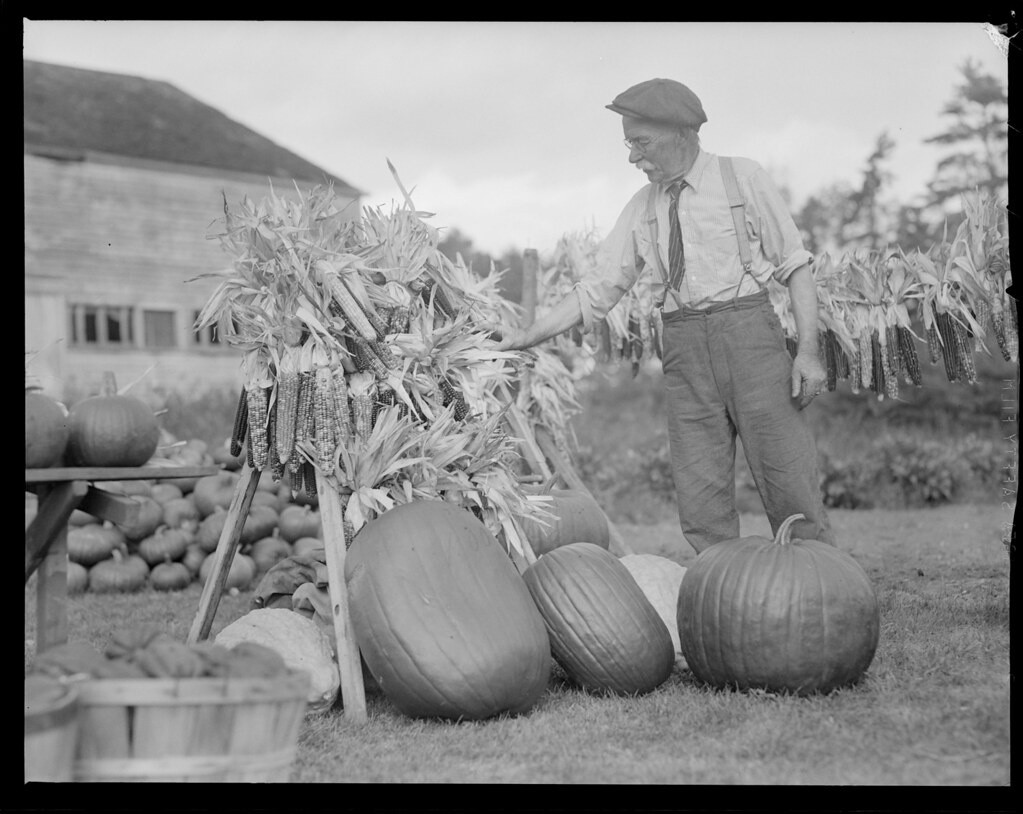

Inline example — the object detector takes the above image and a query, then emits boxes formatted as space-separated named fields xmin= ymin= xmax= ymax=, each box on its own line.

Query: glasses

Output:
xmin=623 ymin=131 xmax=668 ymax=153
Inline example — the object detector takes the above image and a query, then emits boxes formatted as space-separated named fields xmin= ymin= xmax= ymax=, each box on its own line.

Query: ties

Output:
xmin=666 ymin=181 xmax=688 ymax=293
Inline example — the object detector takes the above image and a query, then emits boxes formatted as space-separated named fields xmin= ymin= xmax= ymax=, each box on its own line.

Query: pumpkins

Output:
xmin=24 ymin=373 xmax=161 ymax=470
xmin=677 ymin=512 xmax=880 ymax=694
xmin=67 ymin=429 xmax=322 ymax=591
xmin=344 ymin=467 xmax=676 ymax=721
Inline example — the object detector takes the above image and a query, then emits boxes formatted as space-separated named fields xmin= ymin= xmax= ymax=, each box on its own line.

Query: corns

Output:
xmin=229 ymin=267 xmax=473 ymax=495
xmin=788 ymin=280 xmax=1022 ymax=396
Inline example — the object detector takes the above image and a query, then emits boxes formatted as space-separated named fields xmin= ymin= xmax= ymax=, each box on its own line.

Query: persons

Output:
xmin=472 ymin=79 xmax=832 ymax=555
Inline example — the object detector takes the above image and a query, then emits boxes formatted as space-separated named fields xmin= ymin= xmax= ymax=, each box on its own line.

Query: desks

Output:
xmin=23 ymin=465 xmax=221 ymax=655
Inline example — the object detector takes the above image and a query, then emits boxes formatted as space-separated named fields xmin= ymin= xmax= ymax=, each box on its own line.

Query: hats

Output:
xmin=605 ymin=79 xmax=708 ymax=132
